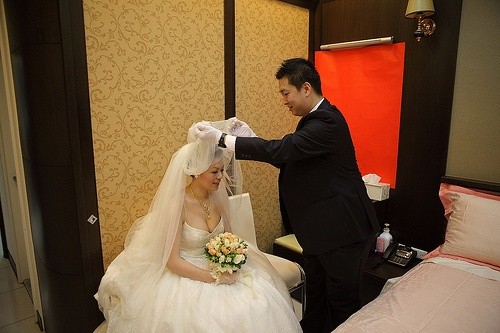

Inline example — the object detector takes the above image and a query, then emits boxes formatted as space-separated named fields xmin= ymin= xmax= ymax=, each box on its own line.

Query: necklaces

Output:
xmin=187 ymin=185 xmax=213 ymax=220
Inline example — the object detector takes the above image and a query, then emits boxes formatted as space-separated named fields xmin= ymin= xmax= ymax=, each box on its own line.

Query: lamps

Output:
xmin=404 ymin=0 xmax=438 ymax=42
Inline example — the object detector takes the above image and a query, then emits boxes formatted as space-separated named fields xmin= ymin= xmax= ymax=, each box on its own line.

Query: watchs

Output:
xmin=217 ymin=133 xmax=228 ymax=148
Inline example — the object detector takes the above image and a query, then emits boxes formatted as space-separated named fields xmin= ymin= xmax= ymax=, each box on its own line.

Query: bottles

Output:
xmin=379 ymin=223 xmax=392 ymax=252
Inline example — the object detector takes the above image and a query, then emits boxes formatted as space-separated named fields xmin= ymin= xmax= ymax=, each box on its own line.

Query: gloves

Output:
xmin=195 ymin=123 xmax=222 ymax=144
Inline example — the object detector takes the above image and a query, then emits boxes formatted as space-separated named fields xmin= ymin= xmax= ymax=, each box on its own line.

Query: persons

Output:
xmin=194 ymin=57 xmax=379 ymax=333
xmin=93 ymin=142 xmax=304 ymax=333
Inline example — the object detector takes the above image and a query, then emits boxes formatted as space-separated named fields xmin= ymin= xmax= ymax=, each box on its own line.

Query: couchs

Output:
xmin=229 ymin=192 xmax=305 ymax=318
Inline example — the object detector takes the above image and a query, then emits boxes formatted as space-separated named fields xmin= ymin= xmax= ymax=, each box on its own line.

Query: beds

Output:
xmin=329 ymin=176 xmax=500 ymax=333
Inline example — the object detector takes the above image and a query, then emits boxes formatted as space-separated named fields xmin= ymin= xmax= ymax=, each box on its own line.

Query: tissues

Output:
xmin=361 ymin=173 xmax=390 ymax=201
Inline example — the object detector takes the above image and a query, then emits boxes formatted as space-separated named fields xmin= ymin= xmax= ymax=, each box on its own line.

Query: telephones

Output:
xmin=382 ymin=240 xmax=417 ymax=267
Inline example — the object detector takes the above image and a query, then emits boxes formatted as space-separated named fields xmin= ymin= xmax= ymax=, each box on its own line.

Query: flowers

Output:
xmin=204 ymin=231 xmax=249 ymax=274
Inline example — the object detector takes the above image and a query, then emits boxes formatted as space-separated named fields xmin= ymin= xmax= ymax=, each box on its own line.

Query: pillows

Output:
xmin=425 ymin=181 xmax=500 ymax=272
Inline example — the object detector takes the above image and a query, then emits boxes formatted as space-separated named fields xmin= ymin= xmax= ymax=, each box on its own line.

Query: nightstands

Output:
xmin=363 ymin=258 xmax=423 ymax=307
